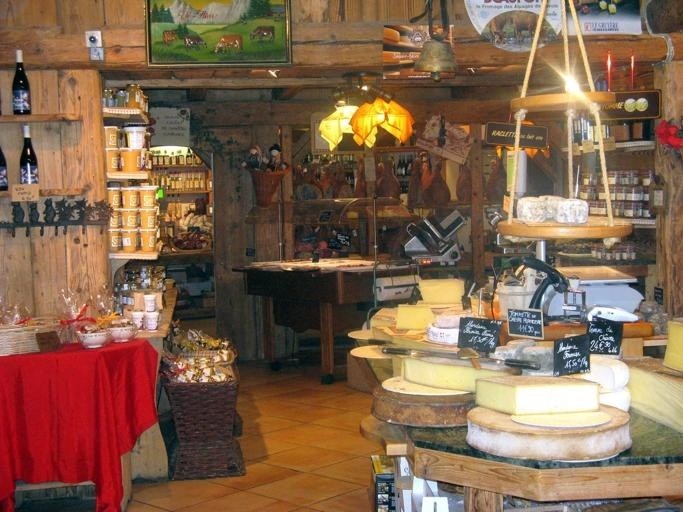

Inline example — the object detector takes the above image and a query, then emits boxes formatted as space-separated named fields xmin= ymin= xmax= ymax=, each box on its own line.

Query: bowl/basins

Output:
xmin=110 ymin=328 xmax=136 ymax=342
xmin=77 ymin=332 xmax=109 ymax=349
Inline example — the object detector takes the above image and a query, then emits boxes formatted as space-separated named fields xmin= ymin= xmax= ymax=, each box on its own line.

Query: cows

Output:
xmin=489 ymin=10 xmax=546 ymax=45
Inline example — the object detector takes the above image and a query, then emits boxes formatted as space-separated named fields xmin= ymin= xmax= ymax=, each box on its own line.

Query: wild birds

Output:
xmin=265 ymin=68 xmax=280 ymax=79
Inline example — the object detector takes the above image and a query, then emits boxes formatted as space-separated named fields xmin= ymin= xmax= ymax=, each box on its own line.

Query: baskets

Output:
xmin=0 ymin=314 xmax=63 ymax=357
xmin=247 ymin=160 xmax=290 ymax=209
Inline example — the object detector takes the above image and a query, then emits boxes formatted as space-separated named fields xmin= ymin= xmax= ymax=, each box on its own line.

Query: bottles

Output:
xmin=166 ymin=194 xmax=183 ymax=238
xmin=302 ymin=154 xmax=417 ymax=194
xmin=11 ymin=49 xmax=32 ymax=115
xmin=19 ymin=125 xmax=39 ymax=185
xmin=148 ymin=147 xmax=206 ymax=192
xmin=103 ymin=83 xmax=149 ymax=116
xmin=0 ymin=145 xmax=8 ymax=192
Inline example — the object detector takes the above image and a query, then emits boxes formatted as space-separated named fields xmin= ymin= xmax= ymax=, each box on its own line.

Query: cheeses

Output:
xmin=372 ymin=280 xmax=465 ymax=344
xmin=489 ymin=334 xmax=631 ymax=412
xmin=663 ymin=321 xmax=683 ymax=372
xmin=475 ymin=374 xmax=601 ymax=415
xmin=403 ymin=354 xmax=501 ymax=391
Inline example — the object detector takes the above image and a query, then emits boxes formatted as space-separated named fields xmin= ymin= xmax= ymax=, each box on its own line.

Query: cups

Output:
xmin=131 ymin=293 xmax=163 ymax=331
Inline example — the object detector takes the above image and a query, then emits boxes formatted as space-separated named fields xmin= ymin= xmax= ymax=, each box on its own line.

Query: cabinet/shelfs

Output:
xmin=150 ymin=163 xmax=213 ymax=258
xmin=559 ymin=138 xmax=657 ymax=278
xmin=0 ymin=113 xmax=84 ymax=197
xmin=102 ymin=106 xmax=178 ymax=339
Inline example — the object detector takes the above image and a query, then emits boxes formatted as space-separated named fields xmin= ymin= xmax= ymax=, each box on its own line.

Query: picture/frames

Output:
xmin=142 ymin=0 xmax=293 ymax=68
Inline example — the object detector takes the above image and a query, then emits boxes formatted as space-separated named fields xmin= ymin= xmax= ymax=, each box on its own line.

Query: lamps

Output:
xmin=314 ymin=69 xmax=415 ymax=153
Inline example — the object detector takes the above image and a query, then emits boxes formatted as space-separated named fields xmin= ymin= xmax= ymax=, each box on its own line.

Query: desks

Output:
xmin=0 ymin=337 xmax=159 ymax=512
xmin=230 ymin=263 xmax=469 ymax=386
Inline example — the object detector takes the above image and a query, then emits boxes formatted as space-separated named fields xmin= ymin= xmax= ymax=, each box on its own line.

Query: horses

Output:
xmin=250 ymin=25 xmax=275 ymax=42
xmin=215 ymin=35 xmax=243 ymax=53
xmin=183 ymin=34 xmax=207 ymax=49
xmin=162 ymin=30 xmax=179 ymax=44
